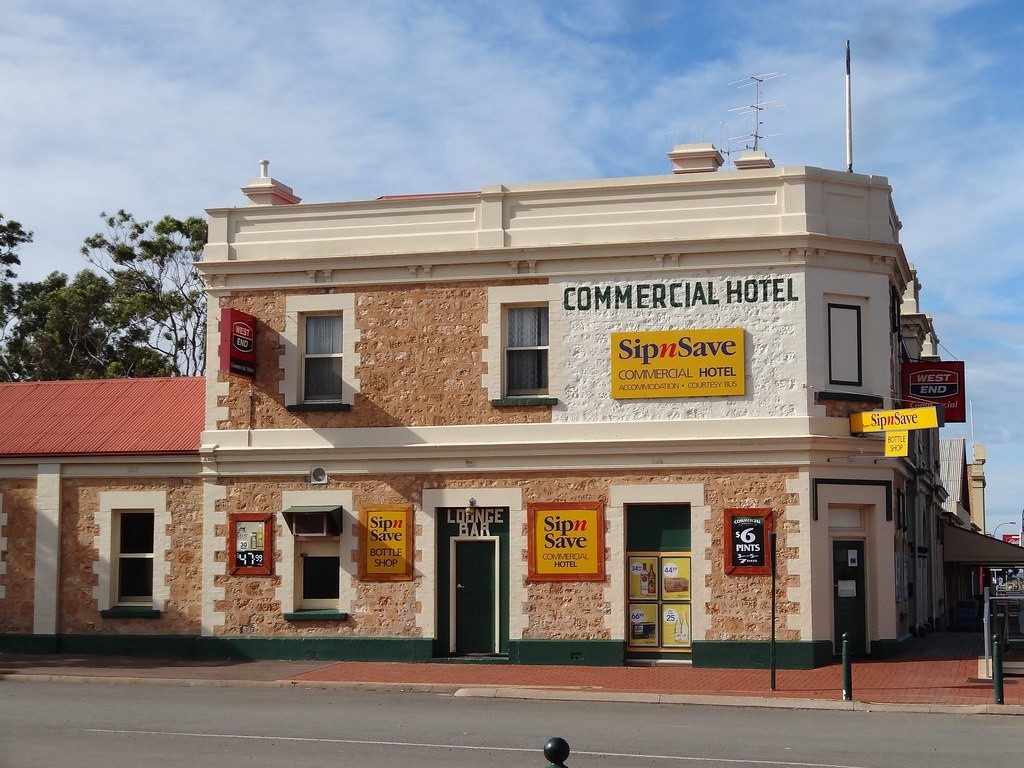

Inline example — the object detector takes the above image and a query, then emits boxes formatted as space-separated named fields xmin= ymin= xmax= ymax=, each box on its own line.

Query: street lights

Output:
xmin=993 ymin=522 xmax=1016 ymax=539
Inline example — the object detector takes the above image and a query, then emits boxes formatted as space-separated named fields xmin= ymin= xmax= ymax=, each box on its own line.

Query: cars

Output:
xmin=1002 ymin=578 xmax=1024 ymax=591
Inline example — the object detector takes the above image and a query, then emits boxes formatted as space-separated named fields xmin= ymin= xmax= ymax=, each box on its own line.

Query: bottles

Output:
xmin=681 ymin=613 xmax=688 ymax=641
xmin=640 ymin=563 xmax=648 ymax=594
xmin=257 ymin=523 xmax=263 ymax=547
xmin=674 ymin=613 xmax=681 ymax=641
xmin=648 ymin=563 xmax=656 ymax=594
xmin=237 ymin=527 xmax=247 ymax=549
xmin=251 ymin=532 xmax=257 ymax=549
